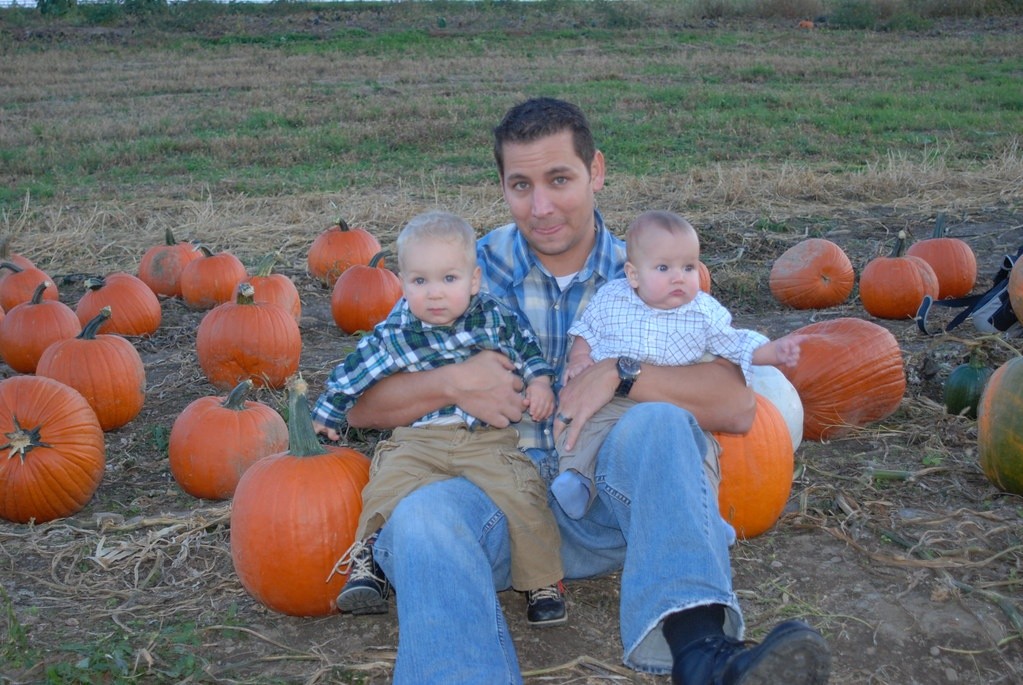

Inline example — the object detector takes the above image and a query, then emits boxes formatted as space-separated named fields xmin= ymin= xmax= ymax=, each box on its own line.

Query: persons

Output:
xmin=550 ymin=211 xmax=802 ymax=545
xmin=311 ymin=212 xmax=567 ymax=629
xmin=371 ymin=96 xmax=832 ymax=684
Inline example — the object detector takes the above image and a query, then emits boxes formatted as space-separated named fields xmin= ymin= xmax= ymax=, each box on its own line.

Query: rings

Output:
xmin=557 ymin=413 xmax=570 ymax=424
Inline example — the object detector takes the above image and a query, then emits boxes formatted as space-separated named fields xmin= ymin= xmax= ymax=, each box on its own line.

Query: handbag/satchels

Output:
xmin=918 ymin=243 xmax=1023 ymax=341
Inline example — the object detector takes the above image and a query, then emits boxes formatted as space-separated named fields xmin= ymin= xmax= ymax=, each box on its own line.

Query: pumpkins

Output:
xmin=0 ymin=216 xmax=404 ymax=615
xmin=696 ymin=209 xmax=1023 ymax=537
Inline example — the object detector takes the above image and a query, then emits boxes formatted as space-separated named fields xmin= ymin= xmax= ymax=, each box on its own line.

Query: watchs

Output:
xmin=615 ymin=356 xmax=641 ymax=398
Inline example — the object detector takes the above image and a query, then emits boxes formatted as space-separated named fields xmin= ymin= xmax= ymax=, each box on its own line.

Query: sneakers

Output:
xmin=668 ymin=620 xmax=832 ymax=685
xmin=526 ymin=581 xmax=569 ymax=629
xmin=325 ymin=534 xmax=389 ymax=615
